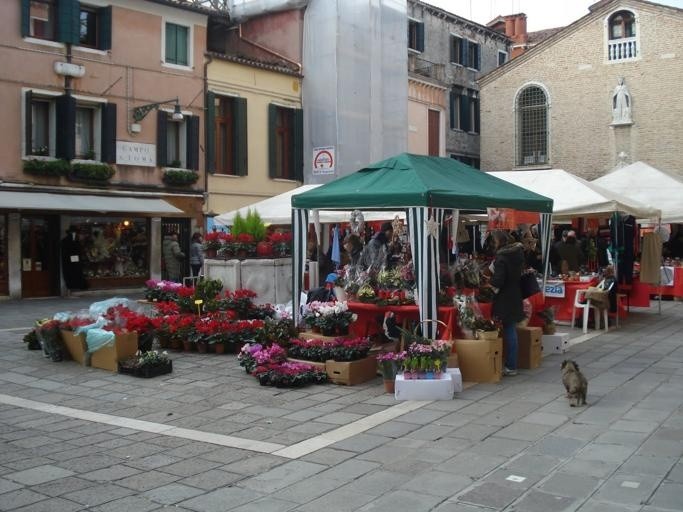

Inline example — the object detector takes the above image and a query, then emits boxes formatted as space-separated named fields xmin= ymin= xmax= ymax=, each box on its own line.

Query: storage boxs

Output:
xmin=514 ymin=322 xmax=543 ymax=372
xmin=541 ymin=332 xmax=570 ymax=357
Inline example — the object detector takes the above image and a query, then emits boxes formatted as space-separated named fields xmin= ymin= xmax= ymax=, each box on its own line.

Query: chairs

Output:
xmin=567 ymin=276 xmax=615 ymax=335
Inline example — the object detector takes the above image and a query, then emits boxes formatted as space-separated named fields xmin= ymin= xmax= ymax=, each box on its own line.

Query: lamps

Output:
xmin=130 ymin=94 xmax=185 ymax=133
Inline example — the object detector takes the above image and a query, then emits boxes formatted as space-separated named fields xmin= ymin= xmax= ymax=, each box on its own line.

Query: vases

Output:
xmin=325 ymin=358 xmax=379 ymax=385
xmin=447 ymin=368 xmax=462 ymax=393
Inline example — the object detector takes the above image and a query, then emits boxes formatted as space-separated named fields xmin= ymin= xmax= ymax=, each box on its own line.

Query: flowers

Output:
xmin=24 ymin=297 xmax=173 ymax=378
xmin=145 ymin=276 xmax=307 ymax=355
xmin=237 ymin=340 xmax=328 ymax=390
xmin=201 ymin=206 xmax=292 ymax=259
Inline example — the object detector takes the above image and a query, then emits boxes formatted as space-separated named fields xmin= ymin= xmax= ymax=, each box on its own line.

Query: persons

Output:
xmin=160 ymin=231 xmax=185 ymax=282
xmin=190 ymin=232 xmax=204 ymax=284
xmin=59 ymin=225 xmax=82 ymax=291
xmin=482 ymin=229 xmax=614 ymax=275
xmin=600 ymin=264 xmax=617 ymax=313
xmin=305 ymin=221 xmax=412 ymax=298
xmin=488 ymin=227 xmax=526 ymax=377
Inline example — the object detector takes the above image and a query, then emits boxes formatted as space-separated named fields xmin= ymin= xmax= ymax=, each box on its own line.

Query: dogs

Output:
xmin=559 ymin=359 xmax=588 ymax=408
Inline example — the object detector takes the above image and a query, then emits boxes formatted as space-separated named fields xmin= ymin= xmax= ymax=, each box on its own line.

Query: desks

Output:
xmin=536 ymin=261 xmax=682 ymax=328
xmin=305 ymin=285 xmax=547 ymax=360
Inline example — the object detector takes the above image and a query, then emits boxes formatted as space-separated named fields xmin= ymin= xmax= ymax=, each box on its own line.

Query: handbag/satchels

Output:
xmin=505 ymin=272 xmax=540 ymax=305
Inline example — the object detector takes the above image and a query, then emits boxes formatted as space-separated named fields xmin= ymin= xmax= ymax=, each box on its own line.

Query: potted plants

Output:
xmin=379 ymin=358 xmax=455 ymax=401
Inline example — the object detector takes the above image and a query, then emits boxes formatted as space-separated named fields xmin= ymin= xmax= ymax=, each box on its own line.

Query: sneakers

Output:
xmin=501 ymin=366 xmax=517 ymax=376
